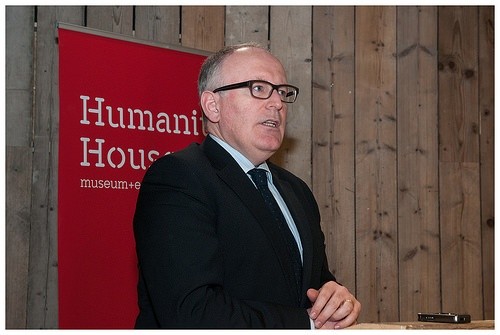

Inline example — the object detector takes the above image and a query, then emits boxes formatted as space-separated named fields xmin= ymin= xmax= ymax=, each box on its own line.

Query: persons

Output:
xmin=133 ymin=44 xmax=361 ymax=330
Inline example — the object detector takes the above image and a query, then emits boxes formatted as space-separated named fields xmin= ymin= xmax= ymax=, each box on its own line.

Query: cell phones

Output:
xmin=418 ymin=312 xmax=471 ymax=324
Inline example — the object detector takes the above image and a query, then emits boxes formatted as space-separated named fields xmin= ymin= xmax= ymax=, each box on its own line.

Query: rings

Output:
xmin=344 ymin=298 xmax=355 ymax=303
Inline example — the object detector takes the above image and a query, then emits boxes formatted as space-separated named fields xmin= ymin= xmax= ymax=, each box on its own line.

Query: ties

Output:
xmin=248 ymin=167 xmax=302 ymax=309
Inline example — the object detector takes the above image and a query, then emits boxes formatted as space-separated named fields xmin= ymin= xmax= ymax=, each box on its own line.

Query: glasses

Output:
xmin=213 ymin=80 xmax=299 ymax=103
